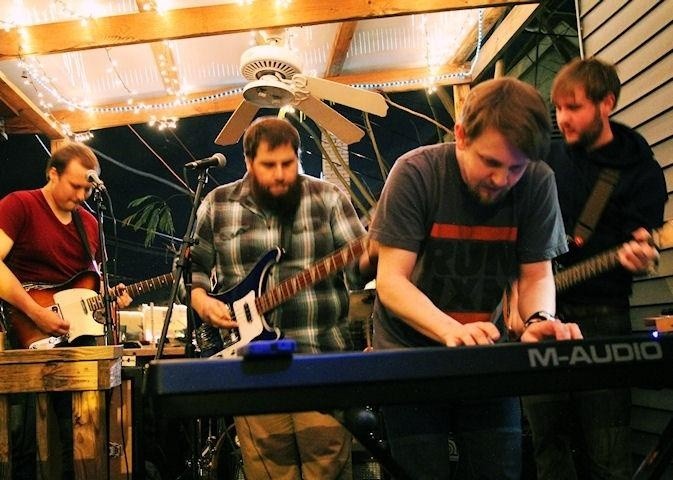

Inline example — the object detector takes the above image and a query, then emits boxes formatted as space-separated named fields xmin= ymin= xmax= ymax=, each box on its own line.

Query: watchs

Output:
xmin=524 ymin=311 xmax=556 ymax=327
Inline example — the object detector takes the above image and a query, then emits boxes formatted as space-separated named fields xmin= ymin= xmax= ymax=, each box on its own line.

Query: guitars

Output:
xmin=0 ymin=270 xmax=181 ymax=350
xmin=503 ymin=229 xmax=661 ymax=333
xmin=185 ymin=232 xmax=374 ymax=358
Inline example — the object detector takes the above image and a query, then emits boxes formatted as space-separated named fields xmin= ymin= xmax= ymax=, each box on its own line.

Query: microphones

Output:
xmin=85 ymin=169 xmax=106 ymax=190
xmin=185 ymin=153 xmax=227 ymax=169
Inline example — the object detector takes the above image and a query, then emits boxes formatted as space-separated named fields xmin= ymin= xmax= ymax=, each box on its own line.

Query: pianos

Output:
xmin=144 ymin=332 xmax=671 ymax=421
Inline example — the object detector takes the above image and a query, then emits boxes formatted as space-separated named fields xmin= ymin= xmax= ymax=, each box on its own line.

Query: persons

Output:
xmin=172 ymin=115 xmax=382 ymax=480
xmin=0 ymin=143 xmax=135 ymax=480
xmin=520 ymin=59 xmax=671 ymax=480
xmin=366 ymin=74 xmax=585 ymax=479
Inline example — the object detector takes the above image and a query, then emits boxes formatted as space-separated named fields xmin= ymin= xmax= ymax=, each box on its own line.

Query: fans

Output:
xmin=213 ymin=38 xmax=389 ymax=147
